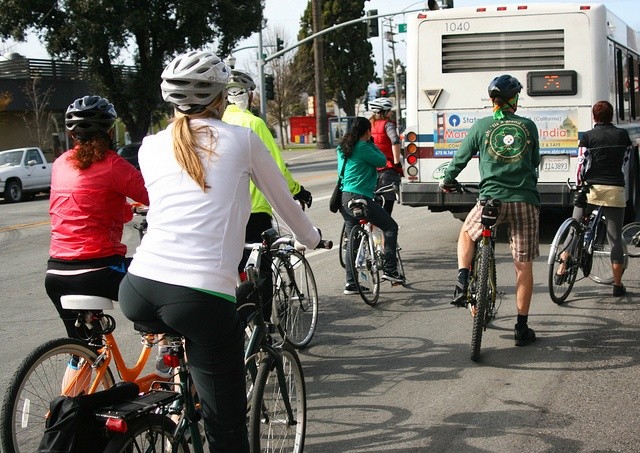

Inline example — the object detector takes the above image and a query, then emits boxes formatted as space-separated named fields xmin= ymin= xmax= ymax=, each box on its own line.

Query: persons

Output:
xmin=220 ymin=71 xmax=312 ymax=323
xmin=554 ymin=100 xmax=633 ymax=297
xmin=438 ymin=75 xmax=541 ymax=346
xmin=118 ymin=51 xmax=322 ymax=453
xmin=368 ymin=96 xmax=405 ymax=217
xmin=336 ymin=117 xmax=403 ymax=295
xmin=45 ymin=96 xmax=149 ymax=420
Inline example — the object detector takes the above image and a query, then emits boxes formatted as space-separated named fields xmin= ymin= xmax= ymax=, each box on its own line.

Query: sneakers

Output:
xmin=514 ymin=324 xmax=535 ymax=346
xmin=381 ymin=271 xmax=407 ymax=282
xmin=343 ymin=282 xmax=371 ymax=295
xmin=450 ymin=292 xmax=468 ymax=306
xmin=555 ymin=271 xmax=569 ymax=286
xmin=613 ymin=285 xmax=627 ymax=297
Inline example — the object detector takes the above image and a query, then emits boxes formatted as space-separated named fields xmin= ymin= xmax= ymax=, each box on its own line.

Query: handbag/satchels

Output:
xmin=329 ymin=142 xmax=348 ymax=213
xmin=38 ymin=382 xmax=138 ymax=453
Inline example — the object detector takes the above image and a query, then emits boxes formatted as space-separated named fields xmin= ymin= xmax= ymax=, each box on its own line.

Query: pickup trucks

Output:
xmin=0 ymin=145 xmax=54 ymax=202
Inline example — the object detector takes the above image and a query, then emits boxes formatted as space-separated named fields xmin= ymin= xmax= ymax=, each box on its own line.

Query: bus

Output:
xmin=402 ymin=3 xmax=638 ymax=212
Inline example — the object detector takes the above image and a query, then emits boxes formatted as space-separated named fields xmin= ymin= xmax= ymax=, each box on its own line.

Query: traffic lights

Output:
xmin=277 ymin=37 xmax=284 ymax=57
xmin=265 ymin=75 xmax=274 ymax=99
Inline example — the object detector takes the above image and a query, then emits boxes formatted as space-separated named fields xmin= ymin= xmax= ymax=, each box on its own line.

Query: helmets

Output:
xmin=65 ymin=94 xmax=118 ymax=135
xmin=161 ymin=50 xmax=229 ymax=114
xmin=489 ymin=75 xmax=522 ymax=100
xmin=225 ymin=67 xmax=257 ymax=91
xmin=368 ymin=98 xmax=393 ymax=113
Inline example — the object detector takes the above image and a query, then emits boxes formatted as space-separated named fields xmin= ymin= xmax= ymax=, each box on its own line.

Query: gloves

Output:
xmin=295 ymin=186 xmax=313 ymax=211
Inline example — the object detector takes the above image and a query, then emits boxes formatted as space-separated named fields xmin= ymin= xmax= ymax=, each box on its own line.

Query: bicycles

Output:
xmin=622 ymin=221 xmax=640 ymax=257
xmin=0 ymin=207 xmax=173 ymax=452
xmin=339 ymin=220 xmax=347 ymax=267
xmin=263 ymin=192 xmax=316 ymax=352
xmin=436 ymin=180 xmax=507 ymax=360
xmin=347 ymin=181 xmax=406 ymax=304
xmin=36 ymin=230 xmax=331 ymax=452
xmin=548 ymin=176 xmax=627 ymax=302
